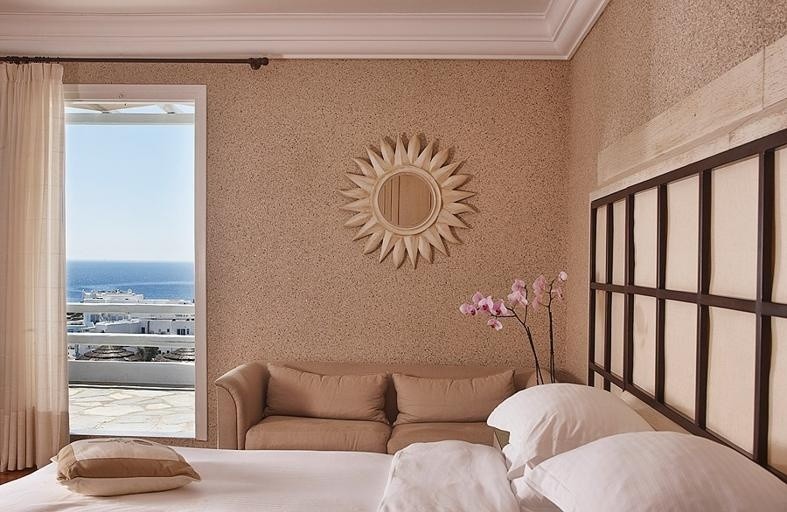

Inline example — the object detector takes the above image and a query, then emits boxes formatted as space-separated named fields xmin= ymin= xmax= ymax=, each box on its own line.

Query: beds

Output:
xmin=0 ymin=390 xmax=786 ymax=512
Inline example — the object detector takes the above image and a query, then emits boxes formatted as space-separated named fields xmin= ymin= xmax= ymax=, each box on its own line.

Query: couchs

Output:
xmin=212 ymin=357 xmax=559 ymax=454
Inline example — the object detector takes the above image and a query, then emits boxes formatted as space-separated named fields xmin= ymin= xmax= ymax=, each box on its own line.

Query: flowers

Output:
xmin=457 ymin=271 xmax=568 ymax=385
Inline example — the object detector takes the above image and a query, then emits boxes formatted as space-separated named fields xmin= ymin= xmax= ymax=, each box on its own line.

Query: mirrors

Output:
xmin=338 ymin=132 xmax=479 ymax=271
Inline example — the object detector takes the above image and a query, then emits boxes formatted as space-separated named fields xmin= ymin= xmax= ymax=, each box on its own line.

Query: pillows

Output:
xmin=50 ymin=437 xmax=201 ymax=497
